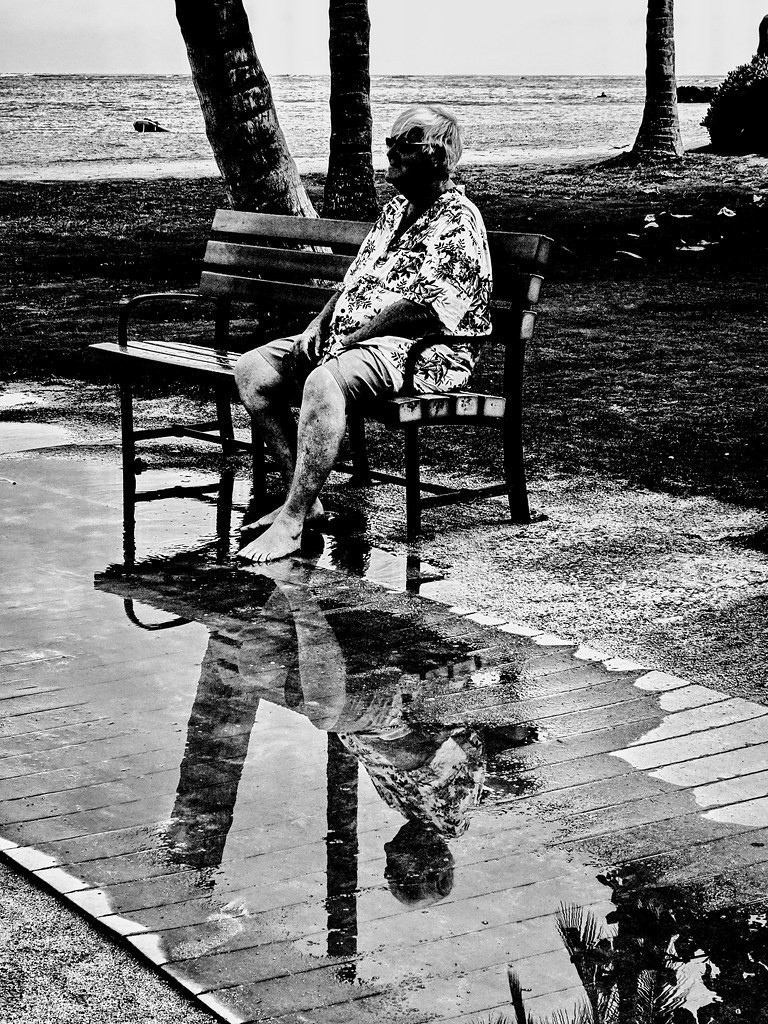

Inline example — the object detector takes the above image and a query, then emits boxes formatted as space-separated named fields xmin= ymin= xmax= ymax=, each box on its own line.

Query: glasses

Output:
xmin=386 ymin=135 xmax=424 ymax=150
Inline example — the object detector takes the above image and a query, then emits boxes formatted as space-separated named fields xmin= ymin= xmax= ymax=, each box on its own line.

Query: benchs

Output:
xmin=86 ymin=211 xmax=551 ymax=538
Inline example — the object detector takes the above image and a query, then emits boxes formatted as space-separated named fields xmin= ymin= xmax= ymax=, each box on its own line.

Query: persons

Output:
xmin=234 ymin=107 xmax=494 ymax=562
